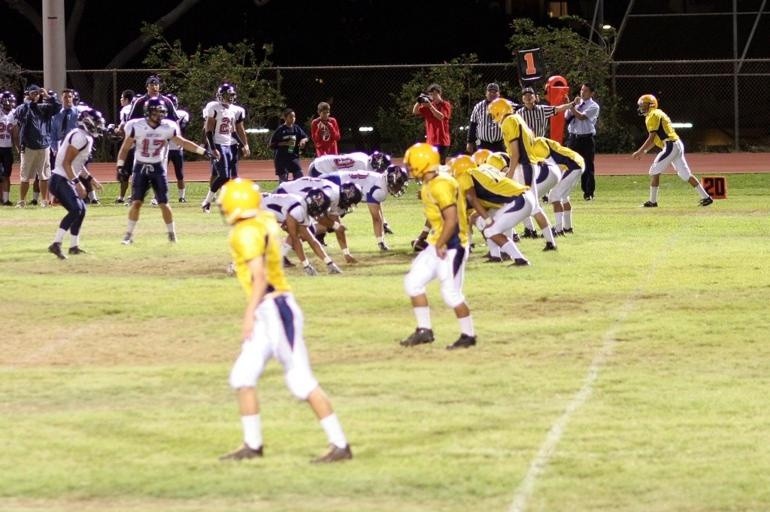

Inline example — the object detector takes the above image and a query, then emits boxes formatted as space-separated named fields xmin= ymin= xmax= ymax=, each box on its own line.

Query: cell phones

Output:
xmin=38 ymin=94 xmax=43 ymax=102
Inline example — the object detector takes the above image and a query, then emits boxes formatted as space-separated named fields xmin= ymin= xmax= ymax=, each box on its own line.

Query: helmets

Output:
xmin=144 ymin=97 xmax=168 ymax=112
xmin=339 ymin=182 xmax=361 ymax=207
xmin=1 ymin=91 xmax=15 ymax=105
xmin=368 ymin=151 xmax=391 ymax=173
xmin=454 ymin=155 xmax=477 ymax=173
xmin=475 ymin=150 xmax=490 ymax=163
xmin=77 ymin=110 xmax=105 ymax=137
xmin=305 ymin=189 xmax=331 ymax=216
xmin=387 ymin=164 xmax=408 ymax=197
xmin=489 ymin=98 xmax=513 ymax=122
xmin=403 ymin=143 xmax=440 ymax=177
xmin=218 ymin=178 xmax=262 ymax=224
xmin=487 ymin=155 xmax=507 ymax=171
xmin=216 ymin=84 xmax=236 ymax=104
xmin=638 ymin=94 xmax=657 ymax=116
xmin=166 ymin=93 xmax=178 ymax=109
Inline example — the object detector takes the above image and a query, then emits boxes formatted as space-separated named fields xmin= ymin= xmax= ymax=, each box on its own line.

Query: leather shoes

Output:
xmin=585 ymin=192 xmax=592 ymax=200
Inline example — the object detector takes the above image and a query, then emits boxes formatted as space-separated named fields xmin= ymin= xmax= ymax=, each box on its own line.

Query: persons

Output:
xmin=12 ymin=86 xmax=61 ymax=208
xmin=514 ymin=87 xmax=581 ymax=138
xmin=50 ymin=90 xmax=102 ymax=201
xmin=449 ymin=156 xmax=535 ymax=267
xmin=564 ymin=83 xmax=599 ymax=200
xmin=202 ymin=84 xmax=248 ymax=212
xmin=161 ymin=94 xmax=189 ymax=203
xmin=276 ymin=176 xmax=362 ymax=264
xmin=466 ymin=83 xmax=512 ymax=155
xmin=309 ymin=102 xmax=340 ymax=156
xmin=271 ymin=108 xmax=308 ymax=188
xmin=630 ymin=95 xmax=713 ymax=208
xmin=49 ymin=108 xmax=106 ymax=260
xmin=319 ymin=165 xmax=409 ymax=250
xmin=529 ymin=130 xmax=585 ymax=236
xmin=415 ymin=86 xmax=452 ymax=158
xmin=216 ymin=177 xmax=351 ymax=462
xmin=252 ymin=190 xmax=338 ymax=274
xmin=132 ymin=77 xmax=175 ymax=119
xmin=401 ymin=144 xmax=477 ymax=351
xmin=490 ymin=102 xmax=559 ymax=251
xmin=108 ymin=92 xmax=143 ymax=205
xmin=310 ymin=150 xmax=395 ymax=235
xmin=1 ymin=91 xmax=20 ymax=205
xmin=115 ymin=96 xmax=216 ymax=248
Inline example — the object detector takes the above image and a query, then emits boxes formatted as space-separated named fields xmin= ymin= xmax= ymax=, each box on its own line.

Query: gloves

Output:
xmin=377 ymin=241 xmax=390 ymax=254
xmin=383 ymin=223 xmax=392 ymax=235
xmin=202 ymin=150 xmax=218 ymax=167
xmin=116 ymin=166 xmax=128 ymax=182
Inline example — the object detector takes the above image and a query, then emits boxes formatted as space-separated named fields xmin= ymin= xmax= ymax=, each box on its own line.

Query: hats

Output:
xmin=487 ymin=83 xmax=500 ymax=91
xmin=147 ymin=76 xmax=160 ymax=84
xmin=522 ymin=88 xmax=535 ymax=94
xmin=28 ymin=85 xmax=41 ymax=93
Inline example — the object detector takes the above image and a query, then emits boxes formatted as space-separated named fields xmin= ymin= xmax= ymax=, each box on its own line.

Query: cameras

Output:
xmin=418 ymin=95 xmax=433 ymax=103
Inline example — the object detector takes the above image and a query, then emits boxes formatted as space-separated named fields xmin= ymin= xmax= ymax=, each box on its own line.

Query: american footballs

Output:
xmin=411 ymin=239 xmax=429 ymax=252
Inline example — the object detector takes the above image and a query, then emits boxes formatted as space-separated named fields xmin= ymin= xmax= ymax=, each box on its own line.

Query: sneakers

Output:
xmin=400 ymin=328 xmax=434 ymax=346
xmin=48 ymin=242 xmax=68 ymax=260
xmin=283 ymin=255 xmax=296 ymax=269
xmin=484 ymin=250 xmax=510 ymax=261
xmin=219 ymin=442 xmax=263 ymax=461
xmin=41 ymin=201 xmax=46 ymax=207
xmin=642 ymin=201 xmax=657 ymax=207
xmin=542 ymin=226 xmax=564 ymax=237
xmin=29 ymin=199 xmax=37 ymax=205
xmin=507 ymin=259 xmax=530 ymax=267
xmin=201 ymin=202 xmax=210 ymax=214
xmin=310 ymin=444 xmax=352 ymax=461
xmin=179 ymin=197 xmax=187 ymax=203
xmin=447 ymin=333 xmax=476 ymax=349
xmin=15 ymin=202 xmax=26 ymax=208
xmin=90 ymin=199 xmax=100 ymax=206
xmin=127 ymin=195 xmax=132 ymax=203
xmin=520 ymin=229 xmax=537 ymax=239
xmin=697 ymin=196 xmax=713 ymax=207
xmin=69 ymin=248 xmax=86 ymax=255
xmin=483 ymin=256 xmax=503 ymax=264
xmin=150 ymin=198 xmax=158 ymax=205
xmin=563 ymin=227 xmax=573 ymax=235
xmin=167 ymin=231 xmax=177 ymax=243
xmin=112 ymin=196 xmax=125 ymax=203
xmin=121 ymin=235 xmax=133 ymax=245
xmin=513 ymin=233 xmax=521 ymax=242
xmin=540 ymin=242 xmax=557 ymax=252
xmin=2 ymin=199 xmax=14 ymax=207
